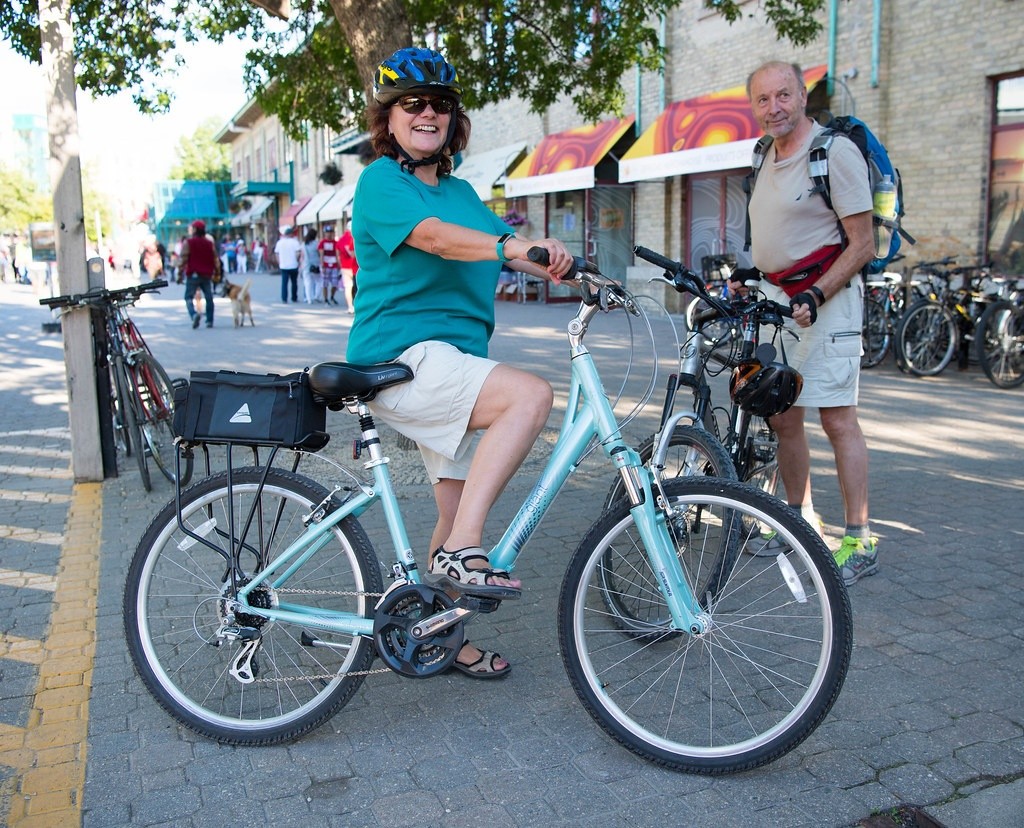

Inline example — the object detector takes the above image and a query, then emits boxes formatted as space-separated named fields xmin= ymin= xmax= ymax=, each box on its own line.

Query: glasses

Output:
xmin=397 ymin=97 xmax=454 ymax=114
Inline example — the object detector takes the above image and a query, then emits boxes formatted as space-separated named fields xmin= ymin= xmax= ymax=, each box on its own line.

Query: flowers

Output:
xmin=500 ymin=209 xmax=529 ymax=226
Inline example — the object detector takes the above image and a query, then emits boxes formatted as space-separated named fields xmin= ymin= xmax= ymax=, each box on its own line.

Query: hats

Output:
xmin=285 ymin=228 xmax=293 ymax=234
xmin=190 ymin=220 xmax=205 ymax=230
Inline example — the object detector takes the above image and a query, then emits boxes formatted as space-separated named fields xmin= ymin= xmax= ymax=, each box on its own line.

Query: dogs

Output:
xmin=220 ymin=278 xmax=256 ymax=329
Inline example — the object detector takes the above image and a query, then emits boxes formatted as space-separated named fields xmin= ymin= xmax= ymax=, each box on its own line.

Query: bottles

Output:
xmin=872 ymin=174 xmax=897 ymax=220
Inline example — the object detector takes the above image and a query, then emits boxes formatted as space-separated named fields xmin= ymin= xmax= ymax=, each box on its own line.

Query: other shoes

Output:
xmin=191 ymin=313 xmax=200 ymax=328
xmin=205 ymin=320 xmax=213 ymax=328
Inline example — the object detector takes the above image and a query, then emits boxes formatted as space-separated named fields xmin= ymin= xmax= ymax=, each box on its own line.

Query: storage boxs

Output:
xmin=495 ymin=284 xmax=538 ymax=301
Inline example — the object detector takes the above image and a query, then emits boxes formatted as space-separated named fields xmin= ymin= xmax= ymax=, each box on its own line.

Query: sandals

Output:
xmin=449 ymin=632 xmax=512 ymax=679
xmin=422 ymin=545 xmax=521 ymax=600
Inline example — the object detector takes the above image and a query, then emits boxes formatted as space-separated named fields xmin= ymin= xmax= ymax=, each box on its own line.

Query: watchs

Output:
xmin=496 ymin=232 xmax=517 ymax=262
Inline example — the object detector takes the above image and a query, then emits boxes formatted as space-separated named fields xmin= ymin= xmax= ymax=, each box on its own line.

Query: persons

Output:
xmin=727 ymin=60 xmax=881 ymax=588
xmin=346 ymin=47 xmax=575 ymax=679
xmin=218 ymin=233 xmax=268 ymax=275
xmin=301 ymin=221 xmax=359 ymax=314
xmin=274 ymin=228 xmax=301 ymax=303
xmin=168 ymin=220 xmax=220 ymax=330
xmin=138 ymin=238 xmax=165 ymax=281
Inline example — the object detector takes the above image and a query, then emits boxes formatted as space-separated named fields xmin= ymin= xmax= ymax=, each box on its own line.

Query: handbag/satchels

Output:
xmin=310 ymin=264 xmax=320 ymax=274
xmin=763 ymin=243 xmax=846 ymax=298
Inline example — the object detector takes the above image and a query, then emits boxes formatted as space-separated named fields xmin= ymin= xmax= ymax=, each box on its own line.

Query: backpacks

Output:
xmin=742 ymin=109 xmax=916 ymax=275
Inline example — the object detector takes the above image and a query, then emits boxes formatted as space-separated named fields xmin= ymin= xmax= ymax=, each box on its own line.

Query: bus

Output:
xmin=26 ymin=223 xmax=59 ymax=284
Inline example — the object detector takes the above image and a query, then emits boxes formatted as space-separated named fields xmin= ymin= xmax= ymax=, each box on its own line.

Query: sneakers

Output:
xmin=746 ymin=519 xmax=824 ymax=556
xmin=832 ymin=535 xmax=880 ymax=586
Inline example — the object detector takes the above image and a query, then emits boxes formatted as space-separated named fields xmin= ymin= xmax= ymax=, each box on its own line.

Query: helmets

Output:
xmin=729 ymin=358 xmax=803 ymax=418
xmin=373 ymin=47 xmax=464 ymax=110
xmin=323 ymin=226 xmax=334 ymax=232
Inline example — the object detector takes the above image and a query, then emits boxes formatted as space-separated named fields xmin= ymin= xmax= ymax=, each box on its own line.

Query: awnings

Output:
xmin=231 ymin=199 xmax=273 ymax=227
xmin=451 ymin=143 xmax=527 ymax=202
xmin=505 ymin=114 xmax=636 ymax=198
xmin=618 ymin=63 xmax=826 ymax=184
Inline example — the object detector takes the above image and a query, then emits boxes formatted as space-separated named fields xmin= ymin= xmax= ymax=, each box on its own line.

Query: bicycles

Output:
xmin=683 ymin=258 xmax=753 ymax=350
xmin=595 ymin=243 xmax=796 ymax=645
xmin=39 ymin=278 xmax=194 ymax=493
xmin=860 ymin=253 xmax=1024 ymax=389
xmin=120 ymin=246 xmax=855 ymax=777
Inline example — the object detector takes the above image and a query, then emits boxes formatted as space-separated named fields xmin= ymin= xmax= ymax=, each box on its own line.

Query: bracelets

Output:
xmin=808 ymin=285 xmax=825 ymax=307
xmin=279 ymin=182 xmax=352 ymax=226
